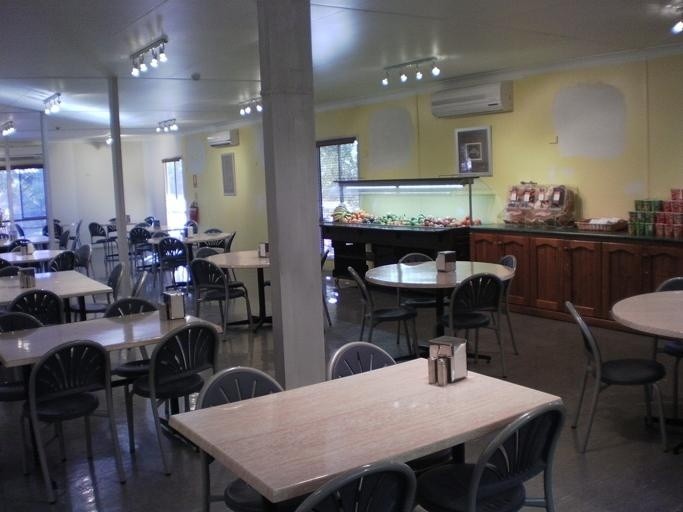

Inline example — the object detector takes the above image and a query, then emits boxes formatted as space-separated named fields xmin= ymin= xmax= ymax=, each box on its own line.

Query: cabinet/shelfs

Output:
xmin=319 ymin=170 xmax=491 ymax=283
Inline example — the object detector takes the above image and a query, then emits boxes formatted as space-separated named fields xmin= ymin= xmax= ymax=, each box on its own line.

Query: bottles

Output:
xmin=436 ymin=354 xmax=447 ymax=385
xmin=427 ymin=357 xmax=436 ymax=385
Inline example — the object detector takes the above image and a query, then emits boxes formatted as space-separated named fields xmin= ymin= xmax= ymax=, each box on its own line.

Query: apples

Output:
xmin=347 ymin=210 xmax=482 ymax=228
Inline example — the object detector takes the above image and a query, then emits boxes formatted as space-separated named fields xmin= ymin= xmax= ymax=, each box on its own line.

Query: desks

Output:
xmin=610 ymin=289 xmax=683 ymax=453
xmin=168 ymin=358 xmax=563 ymax=510
xmin=366 ymin=261 xmax=514 ymax=354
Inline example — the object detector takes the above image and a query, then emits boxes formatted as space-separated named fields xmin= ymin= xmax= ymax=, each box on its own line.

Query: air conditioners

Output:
xmin=429 ymin=80 xmax=514 ymax=119
xmin=206 ymin=128 xmax=240 ymax=147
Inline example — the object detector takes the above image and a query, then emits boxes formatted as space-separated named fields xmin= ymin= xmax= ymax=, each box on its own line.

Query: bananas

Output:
xmin=331 ymin=205 xmax=352 ymax=223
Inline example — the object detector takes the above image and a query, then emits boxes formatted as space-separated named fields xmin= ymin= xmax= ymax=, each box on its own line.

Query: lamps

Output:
xmin=128 ymin=32 xmax=167 ymax=78
xmin=43 ymin=91 xmax=62 ymax=115
xmin=155 ymin=118 xmax=179 ymax=134
xmin=238 ymin=98 xmax=262 ymax=115
xmin=0 ymin=120 xmax=17 ymax=137
xmin=380 ymin=56 xmax=439 ymax=87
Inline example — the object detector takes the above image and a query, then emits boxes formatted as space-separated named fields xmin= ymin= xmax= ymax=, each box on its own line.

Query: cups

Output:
xmin=627 ymin=189 xmax=682 ymax=237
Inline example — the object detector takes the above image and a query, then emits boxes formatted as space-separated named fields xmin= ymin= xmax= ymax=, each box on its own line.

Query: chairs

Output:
xmin=465 ymin=253 xmax=519 ymax=356
xmin=324 ymin=340 xmax=398 ymax=383
xmin=295 ymin=460 xmax=417 ymax=510
xmin=417 ymin=404 xmax=566 ymax=510
xmin=20 ymin=339 xmax=126 ymax=503
xmin=398 ymin=251 xmax=449 ymax=348
xmin=1 ymin=214 xmax=275 ymax=409
xmin=649 ymin=277 xmax=683 ymax=402
xmin=437 ymin=271 xmax=505 ymax=371
xmin=128 ymin=322 xmax=221 ymax=477
xmin=565 ymin=300 xmax=670 ymax=455
xmin=348 ymin=264 xmax=419 ymax=356
xmin=196 ymin=364 xmax=288 ymax=510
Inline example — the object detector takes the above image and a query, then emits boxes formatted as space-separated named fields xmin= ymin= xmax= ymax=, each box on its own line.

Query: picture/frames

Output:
xmin=454 ymin=124 xmax=493 ymax=178
xmin=220 ymin=149 xmax=238 ymax=197
